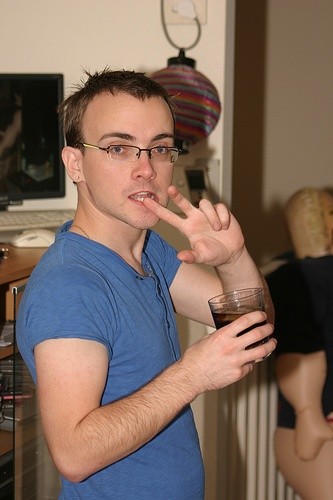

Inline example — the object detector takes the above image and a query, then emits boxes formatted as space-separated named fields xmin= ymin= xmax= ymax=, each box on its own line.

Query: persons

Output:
xmin=14 ymin=68 xmax=278 ymax=500
xmin=262 ymin=186 xmax=333 ymax=500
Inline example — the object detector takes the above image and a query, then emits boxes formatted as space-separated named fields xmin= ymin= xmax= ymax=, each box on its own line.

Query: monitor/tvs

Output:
xmin=0 ymin=72 xmax=66 ymax=210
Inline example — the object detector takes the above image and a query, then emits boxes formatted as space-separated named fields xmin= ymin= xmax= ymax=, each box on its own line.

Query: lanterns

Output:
xmin=149 ymin=55 xmax=221 ymax=158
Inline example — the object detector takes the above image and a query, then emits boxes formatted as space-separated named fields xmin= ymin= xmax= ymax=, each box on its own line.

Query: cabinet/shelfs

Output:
xmin=0 ymin=242 xmax=49 ymax=455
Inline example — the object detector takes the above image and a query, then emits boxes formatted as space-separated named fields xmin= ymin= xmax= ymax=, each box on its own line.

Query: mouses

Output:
xmin=11 ymin=229 xmax=54 ymax=247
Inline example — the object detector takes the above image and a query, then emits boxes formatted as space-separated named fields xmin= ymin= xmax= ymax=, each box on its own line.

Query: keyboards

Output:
xmin=0 ymin=210 xmax=76 ymax=231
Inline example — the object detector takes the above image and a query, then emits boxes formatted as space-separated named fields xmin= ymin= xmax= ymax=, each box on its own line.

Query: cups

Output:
xmin=207 ymin=288 xmax=271 ymax=364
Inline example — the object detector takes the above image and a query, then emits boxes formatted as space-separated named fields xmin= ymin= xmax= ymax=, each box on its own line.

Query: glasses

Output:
xmin=76 ymin=143 xmax=182 ymax=163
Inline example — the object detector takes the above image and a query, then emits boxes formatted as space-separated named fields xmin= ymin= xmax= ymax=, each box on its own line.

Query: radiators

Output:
xmin=203 ymin=358 xmax=301 ymax=500
